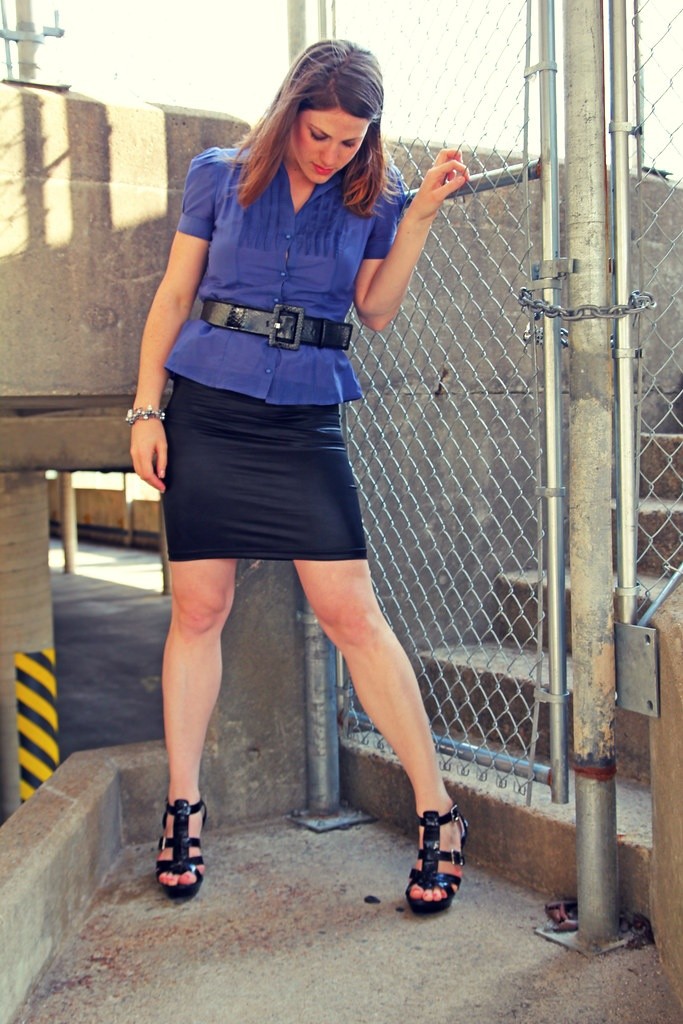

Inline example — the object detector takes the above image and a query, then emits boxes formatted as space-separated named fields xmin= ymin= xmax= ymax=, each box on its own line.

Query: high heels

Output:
xmin=405 ymin=801 xmax=468 ymax=915
xmin=154 ymin=796 xmax=207 ymax=897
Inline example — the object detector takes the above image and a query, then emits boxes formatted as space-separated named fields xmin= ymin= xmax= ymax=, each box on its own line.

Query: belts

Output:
xmin=197 ymin=298 xmax=354 ymax=352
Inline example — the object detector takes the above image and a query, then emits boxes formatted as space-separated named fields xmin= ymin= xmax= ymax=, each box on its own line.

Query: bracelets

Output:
xmin=124 ymin=405 xmax=167 ymax=424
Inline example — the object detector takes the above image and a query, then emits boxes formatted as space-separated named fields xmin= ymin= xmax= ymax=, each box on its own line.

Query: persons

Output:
xmin=119 ymin=40 xmax=471 ymax=916
